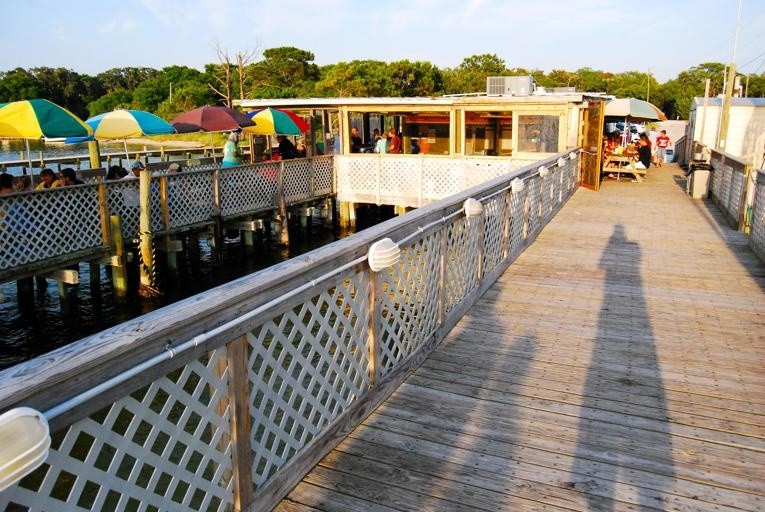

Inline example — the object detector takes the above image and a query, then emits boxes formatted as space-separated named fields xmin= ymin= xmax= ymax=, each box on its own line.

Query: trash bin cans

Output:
xmin=686 ymin=159 xmax=713 ymax=199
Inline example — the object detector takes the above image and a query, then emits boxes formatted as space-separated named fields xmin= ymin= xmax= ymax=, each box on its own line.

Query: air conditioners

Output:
xmin=487 ymin=76 xmax=536 ymax=96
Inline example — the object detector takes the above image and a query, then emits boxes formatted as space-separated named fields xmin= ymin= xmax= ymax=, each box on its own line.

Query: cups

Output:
xmin=693 ymin=153 xmax=703 ymax=160
xmin=614 ymin=144 xmax=633 ymax=155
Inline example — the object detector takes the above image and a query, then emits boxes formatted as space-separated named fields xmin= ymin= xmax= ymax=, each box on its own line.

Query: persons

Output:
xmin=220 ymin=132 xmax=241 ymax=169
xmin=600 ymin=123 xmax=673 ymax=183
xmin=275 ymin=135 xmax=302 ymax=159
xmin=106 ymin=161 xmax=190 ymax=233
xmin=333 ymin=123 xmax=421 ymax=155
xmin=314 ymin=145 xmax=325 ymax=156
xmin=253 ymin=134 xmax=267 ymax=163
xmin=0 ymin=168 xmax=87 ymax=254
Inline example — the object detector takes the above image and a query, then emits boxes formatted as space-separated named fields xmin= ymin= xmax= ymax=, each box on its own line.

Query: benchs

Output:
xmin=600 ymin=168 xmax=646 ymax=183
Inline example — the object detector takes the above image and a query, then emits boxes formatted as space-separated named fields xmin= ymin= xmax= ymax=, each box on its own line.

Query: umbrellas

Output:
xmin=66 ymin=106 xmax=177 ymax=176
xmin=171 ymin=105 xmax=257 ymax=163
xmin=240 ymin=106 xmax=309 ymax=160
xmin=604 ymin=97 xmax=666 ymax=180
xmin=0 ymin=98 xmax=94 ymax=190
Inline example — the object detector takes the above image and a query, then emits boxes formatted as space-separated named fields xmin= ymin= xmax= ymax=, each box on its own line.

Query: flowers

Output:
xmin=526 ymin=130 xmax=543 ymax=152
